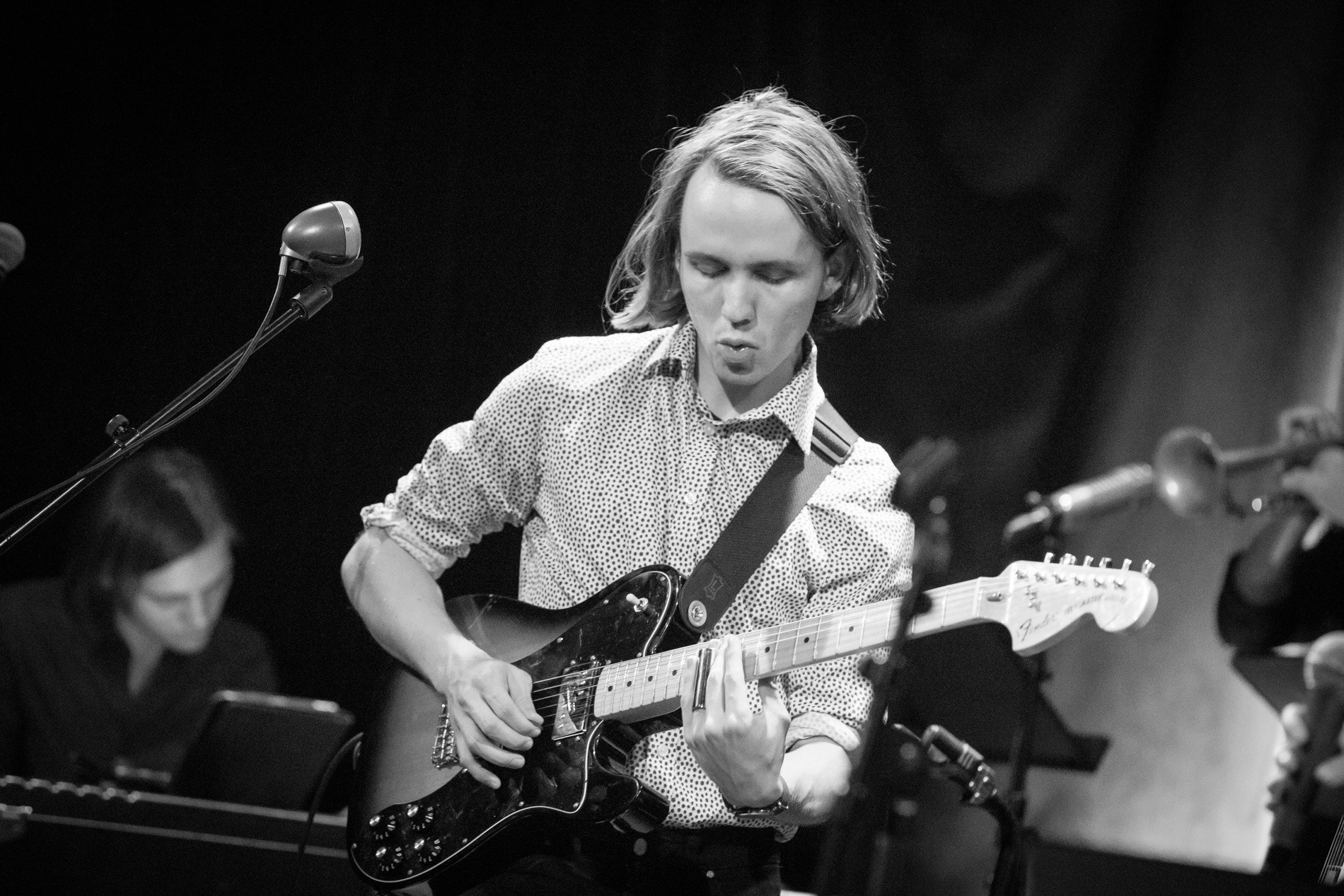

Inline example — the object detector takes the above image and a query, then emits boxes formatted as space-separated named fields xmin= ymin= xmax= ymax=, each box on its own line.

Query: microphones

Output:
xmin=1001 ymin=455 xmax=1161 ymax=548
xmin=280 ymin=200 xmax=363 ymax=261
xmin=0 ymin=221 xmax=27 ymax=280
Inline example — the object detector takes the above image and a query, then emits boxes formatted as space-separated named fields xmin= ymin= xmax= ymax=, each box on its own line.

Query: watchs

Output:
xmin=719 ymin=775 xmax=791 ymax=818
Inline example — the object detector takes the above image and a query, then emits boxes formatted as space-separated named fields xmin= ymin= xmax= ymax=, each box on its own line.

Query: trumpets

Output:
xmin=1147 ymin=418 xmax=1344 ymax=522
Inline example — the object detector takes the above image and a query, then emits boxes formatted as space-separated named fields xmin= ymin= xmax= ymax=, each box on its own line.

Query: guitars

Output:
xmin=343 ymin=549 xmax=1160 ymax=890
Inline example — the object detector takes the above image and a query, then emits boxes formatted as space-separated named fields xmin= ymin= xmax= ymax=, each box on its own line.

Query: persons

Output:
xmin=1214 ymin=319 xmax=1344 ymax=881
xmin=0 ymin=450 xmax=274 ymax=790
xmin=340 ymin=92 xmax=919 ymax=896
